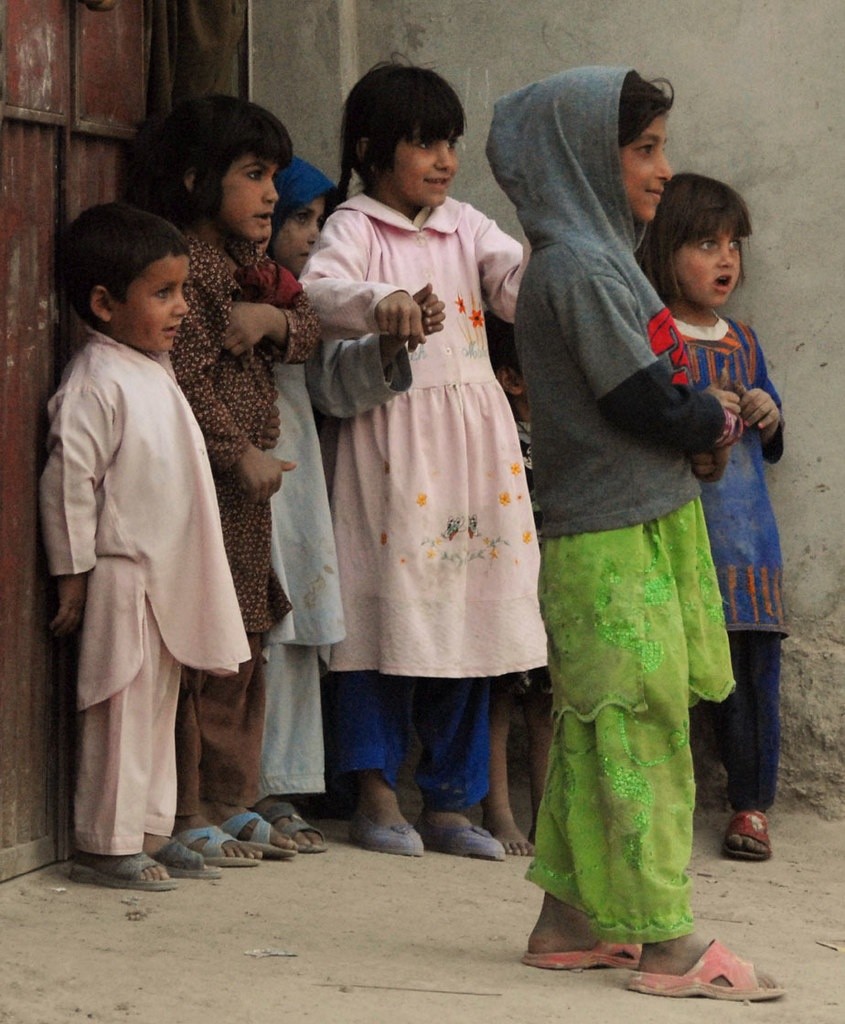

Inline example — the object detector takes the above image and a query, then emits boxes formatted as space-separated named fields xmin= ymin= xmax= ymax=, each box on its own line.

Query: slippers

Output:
xmin=520 ymin=938 xmax=640 ymax=971
xmin=176 ymin=825 xmax=260 ymax=867
xmin=261 ymin=802 xmax=328 ymax=852
xmin=723 ymin=809 xmax=771 ymax=860
xmin=70 ymin=851 xmax=178 ymax=891
xmin=623 ymin=937 xmax=785 ymax=1001
xmin=220 ymin=811 xmax=298 ymax=859
xmin=154 ymin=836 xmax=223 ymax=879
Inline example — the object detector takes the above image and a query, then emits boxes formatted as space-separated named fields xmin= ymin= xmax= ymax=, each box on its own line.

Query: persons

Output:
xmin=150 ymin=51 xmax=789 ymax=866
xmin=483 ymin=65 xmax=790 ymax=1001
xmin=40 ymin=201 xmax=251 ymax=889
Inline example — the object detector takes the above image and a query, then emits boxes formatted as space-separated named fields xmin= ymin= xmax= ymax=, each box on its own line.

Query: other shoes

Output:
xmin=415 ymin=810 xmax=506 ymax=860
xmin=348 ymin=809 xmax=425 ymax=856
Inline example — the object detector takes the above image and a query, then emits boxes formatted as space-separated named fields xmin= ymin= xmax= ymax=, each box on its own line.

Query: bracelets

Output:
xmin=715 ymin=405 xmax=743 ymax=449
